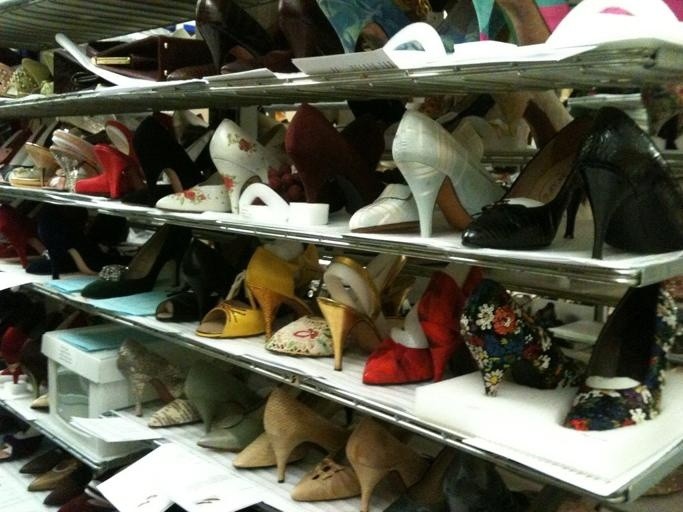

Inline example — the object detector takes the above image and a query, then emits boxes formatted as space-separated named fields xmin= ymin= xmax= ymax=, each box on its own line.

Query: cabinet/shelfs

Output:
xmin=1 ymin=0 xmax=683 ymax=512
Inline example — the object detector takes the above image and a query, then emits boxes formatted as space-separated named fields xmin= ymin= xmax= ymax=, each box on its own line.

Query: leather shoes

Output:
xmin=19 ymin=447 xmax=115 ymax=512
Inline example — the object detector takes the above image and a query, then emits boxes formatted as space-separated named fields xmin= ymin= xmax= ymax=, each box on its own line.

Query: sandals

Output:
xmin=0 ymin=421 xmax=44 ymax=462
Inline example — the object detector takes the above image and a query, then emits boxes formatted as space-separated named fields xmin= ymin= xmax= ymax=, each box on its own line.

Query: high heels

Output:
xmin=1 ymin=0 xmax=683 ymax=436
xmin=197 ymin=388 xmax=538 ymax=512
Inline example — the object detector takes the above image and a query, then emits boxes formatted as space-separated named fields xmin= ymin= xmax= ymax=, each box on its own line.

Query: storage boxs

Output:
xmin=40 ymin=322 xmax=214 ymax=458
